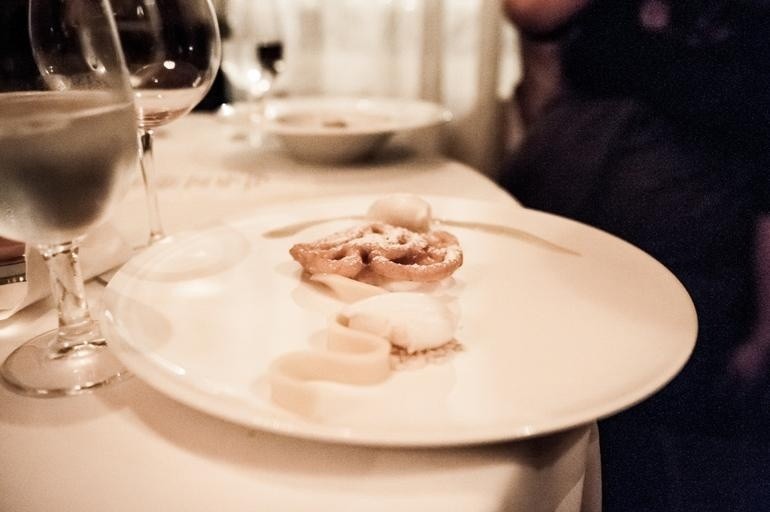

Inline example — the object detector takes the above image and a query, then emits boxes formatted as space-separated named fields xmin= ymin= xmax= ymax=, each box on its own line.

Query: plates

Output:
xmin=223 ymin=97 xmax=453 ymax=162
xmin=92 ymin=195 xmax=700 ymax=448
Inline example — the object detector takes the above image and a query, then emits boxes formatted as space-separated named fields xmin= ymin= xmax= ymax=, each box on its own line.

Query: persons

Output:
xmin=487 ymin=1 xmax=769 ymax=512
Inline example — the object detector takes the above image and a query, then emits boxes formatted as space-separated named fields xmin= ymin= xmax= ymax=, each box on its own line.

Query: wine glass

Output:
xmin=222 ymin=32 xmax=291 ymax=164
xmin=0 ymin=84 xmax=139 ymax=394
xmin=30 ymin=0 xmax=226 ymax=246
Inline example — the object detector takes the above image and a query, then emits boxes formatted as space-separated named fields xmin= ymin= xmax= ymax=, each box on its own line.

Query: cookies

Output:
xmin=290 ymin=220 xmax=463 ymax=281
